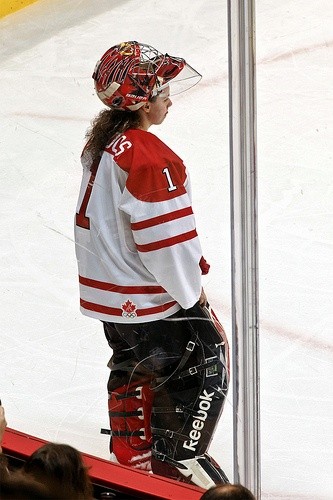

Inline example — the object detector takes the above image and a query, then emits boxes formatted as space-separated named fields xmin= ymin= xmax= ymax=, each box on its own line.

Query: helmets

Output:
xmin=92 ymin=41 xmax=186 ymax=112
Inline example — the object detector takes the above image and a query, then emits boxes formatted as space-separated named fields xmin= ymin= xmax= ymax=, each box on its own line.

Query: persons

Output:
xmin=200 ymin=485 xmax=256 ymax=500
xmin=73 ymin=40 xmax=229 ymax=487
xmin=0 ymin=400 xmax=94 ymax=500
